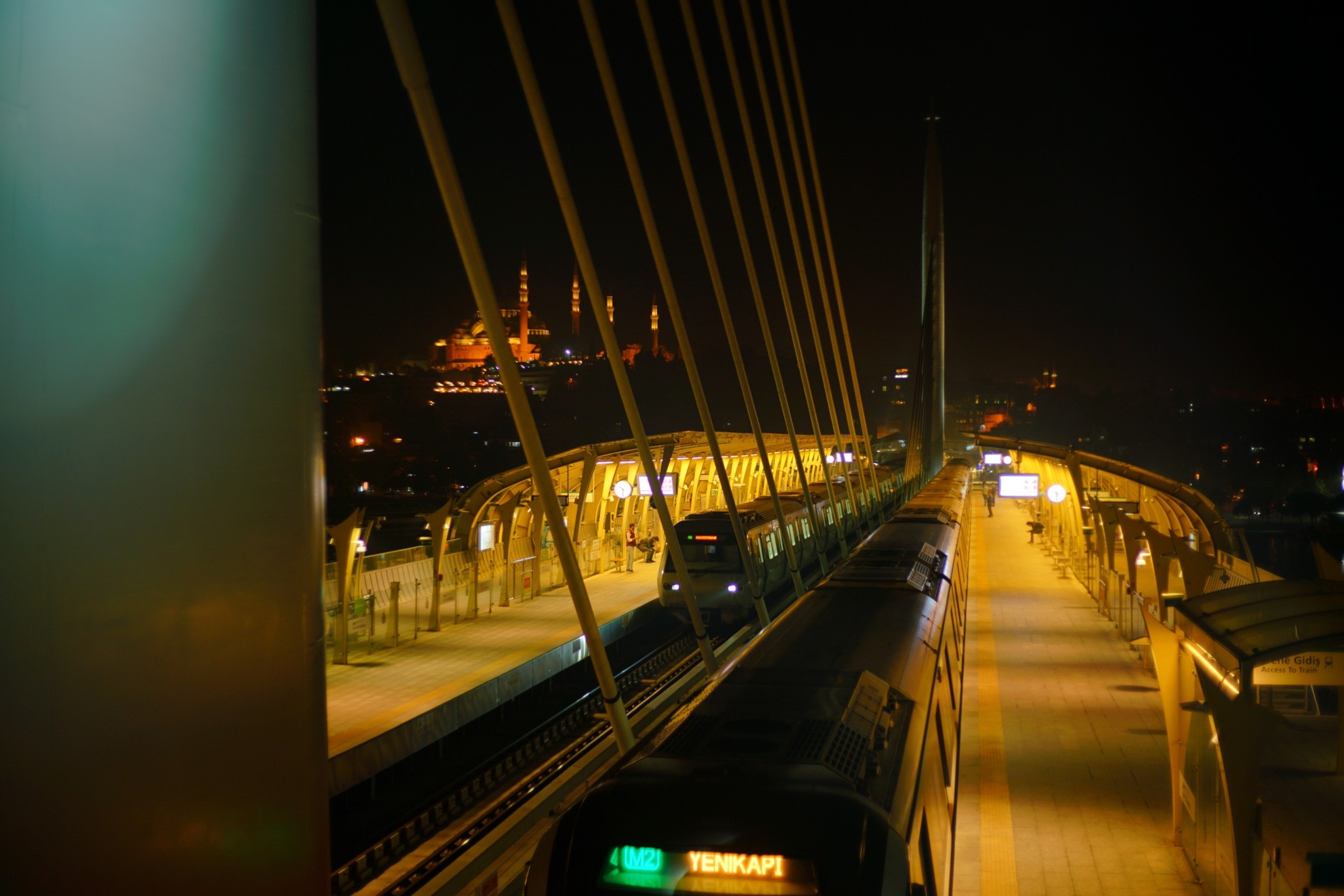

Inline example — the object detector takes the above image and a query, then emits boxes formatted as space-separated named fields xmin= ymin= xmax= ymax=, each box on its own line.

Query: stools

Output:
xmin=639 ymin=549 xmax=656 ymax=563
xmin=1036 ymin=531 xmax=1072 ymax=580
xmin=1015 ymin=499 xmax=1028 ymax=513
xmin=611 ymin=558 xmax=627 ymax=571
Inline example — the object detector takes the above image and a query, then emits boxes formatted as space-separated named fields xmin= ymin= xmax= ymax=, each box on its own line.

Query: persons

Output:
xmin=636 ymin=536 xmax=660 ymax=564
xmin=626 ymin=523 xmax=640 ymax=573
xmin=986 ymin=491 xmax=994 ymax=517
xmin=982 ymin=482 xmax=997 ymax=506
xmin=1026 ymin=521 xmax=1045 ymax=544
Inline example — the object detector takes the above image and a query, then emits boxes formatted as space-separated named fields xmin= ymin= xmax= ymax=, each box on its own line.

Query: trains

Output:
xmin=520 ymin=456 xmax=973 ymax=896
xmin=657 ymin=455 xmax=927 ymax=610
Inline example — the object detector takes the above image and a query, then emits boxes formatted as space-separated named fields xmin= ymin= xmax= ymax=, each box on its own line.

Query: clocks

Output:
xmin=614 ymin=481 xmax=632 ymax=499
xmin=1047 ymin=484 xmax=1066 ymax=503
